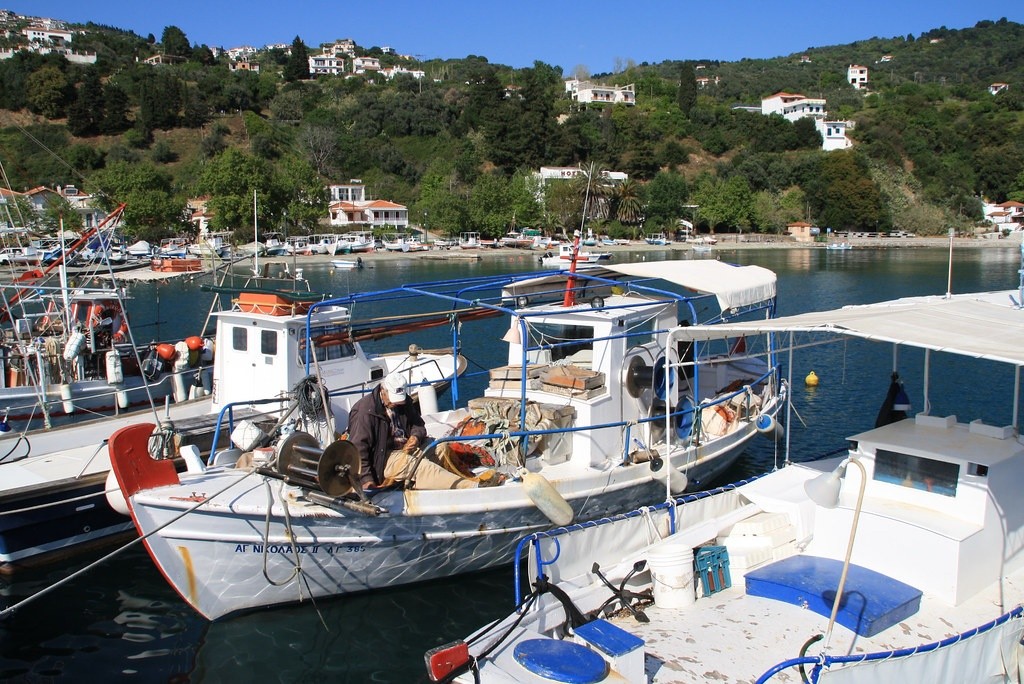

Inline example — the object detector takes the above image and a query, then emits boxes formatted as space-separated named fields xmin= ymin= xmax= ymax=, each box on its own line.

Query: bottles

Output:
xmin=418 ymin=377 xmax=438 ymax=414
xmin=519 ymin=468 xmax=574 ymax=526
xmin=756 ymin=414 xmax=784 ymax=440
xmin=650 ymin=457 xmax=687 ymax=493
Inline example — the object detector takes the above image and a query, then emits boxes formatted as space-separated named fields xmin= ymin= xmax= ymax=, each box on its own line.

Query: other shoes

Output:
xmin=479 ymin=473 xmax=507 ymax=488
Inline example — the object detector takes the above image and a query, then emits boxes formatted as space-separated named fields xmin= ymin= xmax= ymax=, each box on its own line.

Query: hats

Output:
xmin=383 ymin=372 xmax=407 ymax=404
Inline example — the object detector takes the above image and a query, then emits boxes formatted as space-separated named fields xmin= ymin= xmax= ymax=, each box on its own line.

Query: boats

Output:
xmin=826 ymin=242 xmax=854 ymax=250
xmin=645 ymin=237 xmax=673 ymax=246
xmin=541 ymin=242 xmax=613 ymax=266
xmin=106 ymin=164 xmax=793 ymax=621
xmin=331 ymin=258 xmax=363 ymax=270
xmin=686 ymin=235 xmax=719 ymax=244
xmin=0 ymin=208 xmax=633 ymax=266
xmin=0 ymin=191 xmax=469 ymax=574
xmin=421 ymin=225 xmax=1024 ymax=684
xmin=0 ymin=110 xmax=218 ymax=444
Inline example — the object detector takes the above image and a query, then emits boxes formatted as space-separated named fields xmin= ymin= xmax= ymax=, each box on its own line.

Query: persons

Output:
xmin=201 ymin=328 xmax=216 ymax=364
xmin=349 ymin=373 xmax=508 ymax=490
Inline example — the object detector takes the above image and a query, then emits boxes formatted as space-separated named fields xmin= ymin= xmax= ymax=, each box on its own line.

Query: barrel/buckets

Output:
xmin=647 ymin=543 xmax=698 ymax=609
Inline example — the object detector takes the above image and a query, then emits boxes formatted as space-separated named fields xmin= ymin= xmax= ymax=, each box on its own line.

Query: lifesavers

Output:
xmin=85 ymin=300 xmax=129 ymax=343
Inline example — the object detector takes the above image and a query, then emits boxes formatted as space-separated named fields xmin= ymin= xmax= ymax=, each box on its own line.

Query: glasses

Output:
xmin=386 ymin=394 xmax=406 ymax=405
